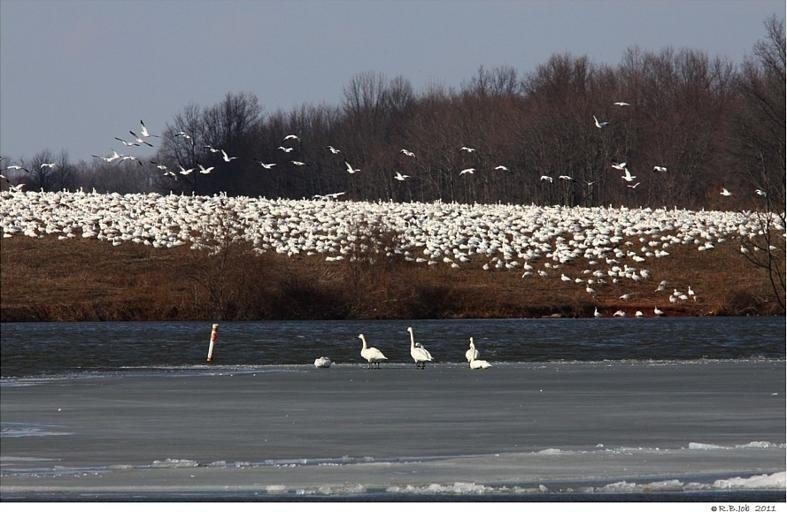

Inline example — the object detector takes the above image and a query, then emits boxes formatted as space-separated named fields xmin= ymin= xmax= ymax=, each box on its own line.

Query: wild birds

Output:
xmin=469 ymin=343 xmax=493 ymax=370
xmin=354 ymin=333 xmax=385 ymax=369
xmin=313 ymin=356 xmax=332 ymax=368
xmin=592 ymin=115 xmax=610 ymax=128
xmin=465 ymin=337 xmax=480 ymax=363
xmin=369 ymin=347 xmax=389 ymax=369
xmin=0 ymin=120 xmax=786 ymax=318
xmin=406 ymin=327 xmax=432 ymax=369
xmin=415 ymin=342 xmax=435 ymax=367
xmin=613 ymin=102 xmax=631 ymax=106
xmin=344 ymin=158 xmax=360 ymax=174
